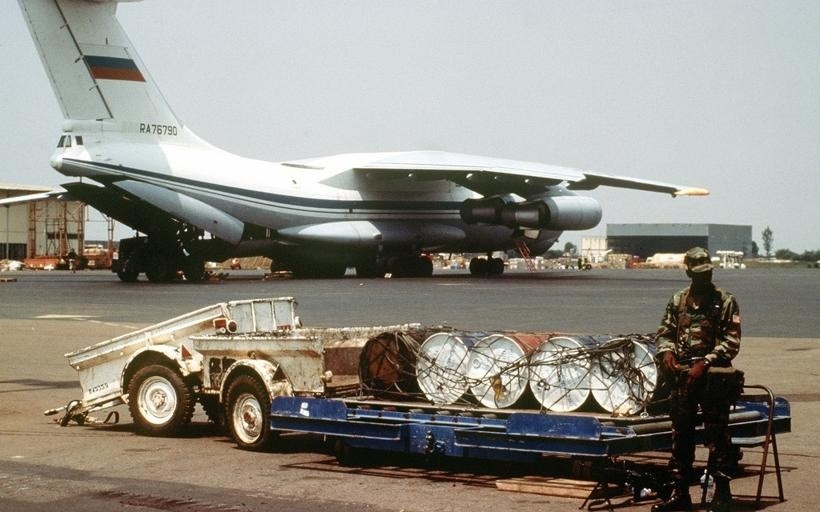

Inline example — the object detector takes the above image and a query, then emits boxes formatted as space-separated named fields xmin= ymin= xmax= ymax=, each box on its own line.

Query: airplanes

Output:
xmin=14 ymin=1 xmax=711 ymax=283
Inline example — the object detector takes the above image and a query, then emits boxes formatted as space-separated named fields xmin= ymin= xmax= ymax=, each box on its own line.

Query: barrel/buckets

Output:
xmin=589 ymin=329 xmax=675 ymax=416
xmin=357 ymin=325 xmax=453 ymax=399
xmin=414 ymin=328 xmax=495 ymax=406
xmin=467 ymin=328 xmax=564 ymax=409
xmin=528 ymin=332 xmax=618 ymax=411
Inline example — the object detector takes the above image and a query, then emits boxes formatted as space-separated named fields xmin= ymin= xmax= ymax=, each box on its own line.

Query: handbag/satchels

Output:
xmin=707 ymin=365 xmax=745 ymax=398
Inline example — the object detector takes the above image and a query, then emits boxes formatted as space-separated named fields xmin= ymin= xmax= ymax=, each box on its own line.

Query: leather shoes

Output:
xmin=707 ymin=493 xmax=733 ymax=512
xmin=651 ymin=494 xmax=692 ymax=512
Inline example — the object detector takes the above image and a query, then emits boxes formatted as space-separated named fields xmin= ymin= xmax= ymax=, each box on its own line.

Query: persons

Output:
xmin=66 ymin=248 xmax=81 ymax=273
xmin=649 ymin=245 xmax=745 ymax=512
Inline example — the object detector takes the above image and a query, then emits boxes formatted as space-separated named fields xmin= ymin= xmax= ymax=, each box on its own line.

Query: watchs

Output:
xmin=703 ymin=358 xmax=710 ymax=366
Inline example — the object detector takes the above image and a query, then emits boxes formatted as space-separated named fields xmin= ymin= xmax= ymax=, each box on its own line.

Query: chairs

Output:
xmin=702 ymin=384 xmax=784 ymax=509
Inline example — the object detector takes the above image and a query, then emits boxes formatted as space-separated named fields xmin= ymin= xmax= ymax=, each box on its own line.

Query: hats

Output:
xmin=685 ymin=247 xmax=713 ymax=273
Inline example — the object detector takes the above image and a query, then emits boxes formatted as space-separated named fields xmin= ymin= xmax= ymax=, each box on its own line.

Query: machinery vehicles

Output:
xmin=45 ymin=289 xmax=428 ymax=453
